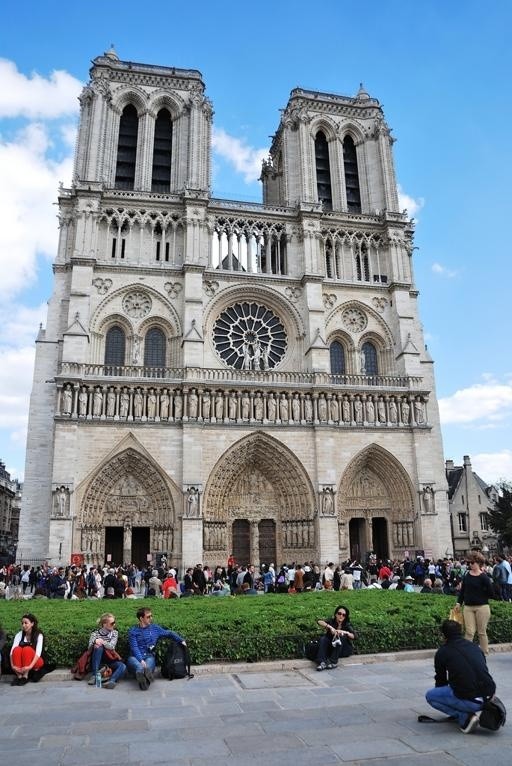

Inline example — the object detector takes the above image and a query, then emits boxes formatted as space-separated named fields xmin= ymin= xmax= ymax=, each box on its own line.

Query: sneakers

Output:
xmin=457 ymin=713 xmax=480 ymax=735
xmin=316 ymin=661 xmax=339 ymax=671
xmin=17 ymin=676 xmax=29 ymax=686
xmin=87 ymin=674 xmax=97 ymax=685
xmin=100 ymin=679 xmax=116 ymax=690
xmin=10 ymin=676 xmax=19 ymax=686
xmin=134 ymin=666 xmax=156 ymax=691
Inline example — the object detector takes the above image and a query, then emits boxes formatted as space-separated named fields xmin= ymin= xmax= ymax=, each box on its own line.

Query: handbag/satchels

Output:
xmin=478 ymin=694 xmax=507 ymax=732
xmin=104 ymin=646 xmax=122 ymax=662
xmin=304 ymin=639 xmax=321 ymax=661
xmin=449 ymin=609 xmax=465 ymax=625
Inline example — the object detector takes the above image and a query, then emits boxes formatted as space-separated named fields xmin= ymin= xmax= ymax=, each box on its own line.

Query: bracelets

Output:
xmin=325 ymin=623 xmax=328 ymax=628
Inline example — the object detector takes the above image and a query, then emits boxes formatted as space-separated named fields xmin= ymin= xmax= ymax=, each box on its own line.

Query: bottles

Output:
xmin=95 ymin=671 xmax=102 ymax=689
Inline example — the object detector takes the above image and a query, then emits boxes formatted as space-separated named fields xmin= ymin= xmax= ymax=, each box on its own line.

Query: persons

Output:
xmin=0 ymin=553 xmax=512 ymax=601
xmin=87 ymin=612 xmax=127 ymax=689
xmin=56 ymin=488 xmax=66 ymax=516
xmin=423 ymin=488 xmax=434 ymax=512
xmin=187 ymin=488 xmax=198 ymax=516
xmin=453 ymin=550 xmax=493 ymax=658
xmin=61 ymin=383 xmax=426 ymax=425
xmin=315 ymin=605 xmax=359 ymax=671
xmin=9 ymin=613 xmax=49 ymax=686
xmin=322 ymin=489 xmax=333 ymax=514
xmin=425 ymin=619 xmax=496 ymax=735
xmin=126 ymin=606 xmax=187 ymax=690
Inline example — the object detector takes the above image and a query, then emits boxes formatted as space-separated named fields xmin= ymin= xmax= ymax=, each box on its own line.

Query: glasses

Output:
xmin=466 ymin=561 xmax=477 ymax=565
xmin=106 ymin=621 xmax=115 ymax=626
xmin=336 ymin=611 xmax=346 ymax=617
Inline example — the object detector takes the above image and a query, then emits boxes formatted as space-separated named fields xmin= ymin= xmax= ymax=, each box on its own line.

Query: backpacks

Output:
xmin=161 ymin=640 xmax=192 ymax=682
